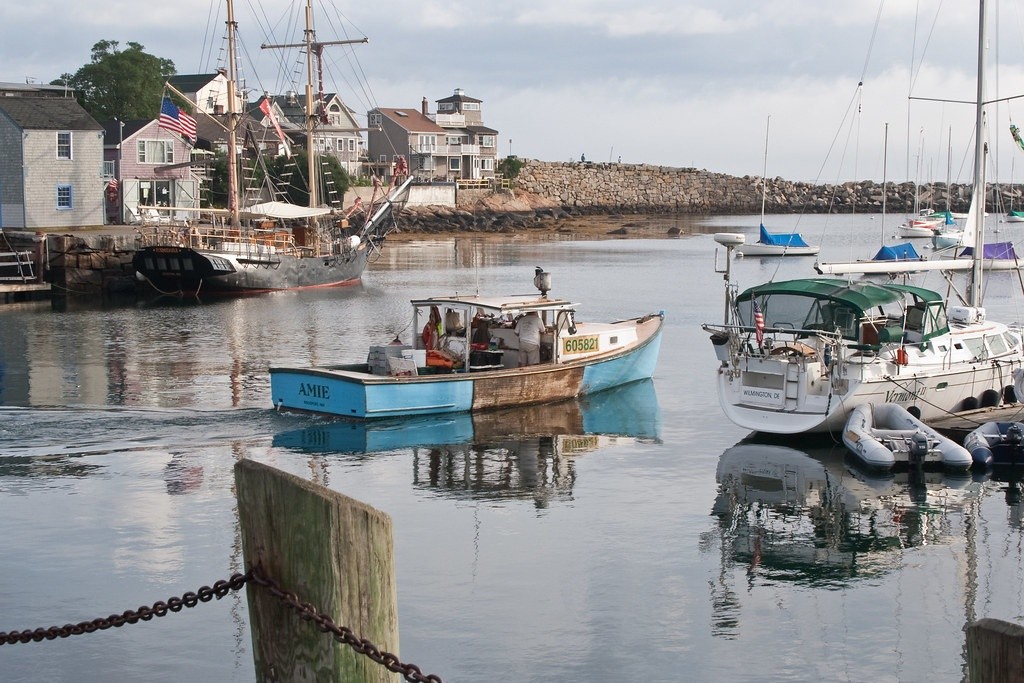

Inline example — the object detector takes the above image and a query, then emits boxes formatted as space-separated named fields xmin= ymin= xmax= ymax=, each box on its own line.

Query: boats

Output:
xmin=963 ymin=420 xmax=1024 ymax=468
xmin=269 ymin=379 xmax=664 ymax=516
xmin=268 ymin=265 xmax=666 ymax=420
xmin=843 ymin=402 xmax=973 ymax=474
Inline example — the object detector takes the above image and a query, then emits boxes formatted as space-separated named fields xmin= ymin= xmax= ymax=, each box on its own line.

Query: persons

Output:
xmin=618 ymin=156 xmax=621 ymax=163
xmin=581 ymin=153 xmax=585 ymax=161
xmin=514 ymin=312 xmax=545 ymax=368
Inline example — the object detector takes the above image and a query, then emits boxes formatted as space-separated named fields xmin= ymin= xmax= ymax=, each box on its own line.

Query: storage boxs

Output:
xmin=477 ymin=350 xmax=504 ymax=365
xmin=427 ymin=350 xmax=454 ymax=367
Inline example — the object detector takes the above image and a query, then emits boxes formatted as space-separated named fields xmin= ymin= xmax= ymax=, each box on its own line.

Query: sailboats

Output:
xmin=710 ymin=428 xmax=1002 ymax=682
xmin=131 ymin=1 xmax=414 ymax=295
xmin=697 ymin=0 xmax=1024 ymax=436
xmin=733 ymin=114 xmax=821 ymax=256
xmin=856 ymin=122 xmax=1024 ymax=274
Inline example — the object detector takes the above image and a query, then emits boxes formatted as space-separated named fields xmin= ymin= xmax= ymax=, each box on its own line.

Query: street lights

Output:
xmin=119 ymin=120 xmax=126 ymax=225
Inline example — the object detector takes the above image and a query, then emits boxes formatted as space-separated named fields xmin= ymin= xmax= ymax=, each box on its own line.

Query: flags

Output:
xmin=753 ymin=295 xmax=764 ymax=341
xmin=1010 ymin=125 xmax=1024 ymax=149
xmin=259 ymin=99 xmax=291 ymax=160
xmin=158 ymin=97 xmax=197 ymax=143
